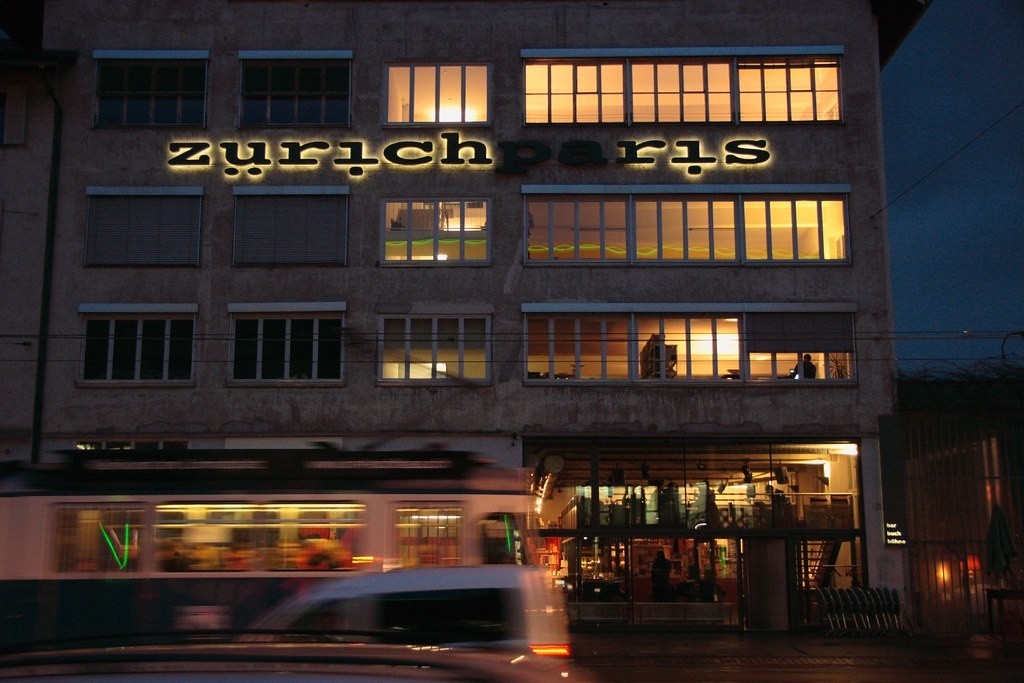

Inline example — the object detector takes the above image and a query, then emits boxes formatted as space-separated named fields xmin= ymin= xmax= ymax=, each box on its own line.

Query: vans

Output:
xmin=230 ymin=565 xmax=569 ymax=662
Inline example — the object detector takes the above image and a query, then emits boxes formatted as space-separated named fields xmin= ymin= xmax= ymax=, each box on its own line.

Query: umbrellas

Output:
xmin=987 ymin=504 xmax=1018 ymax=589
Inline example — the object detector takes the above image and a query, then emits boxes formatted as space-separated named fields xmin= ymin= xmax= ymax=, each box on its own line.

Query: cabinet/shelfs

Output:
xmin=642 ymin=333 xmax=677 ymax=379
xmin=550 ymin=479 xmax=765 ymax=627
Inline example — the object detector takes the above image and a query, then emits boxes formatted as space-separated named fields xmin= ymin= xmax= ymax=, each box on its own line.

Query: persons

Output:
xmin=651 ymin=551 xmax=672 ymax=602
xmin=661 ymin=481 xmax=679 ymax=529
xmin=789 ymin=354 xmax=817 ymax=379
xmin=765 ymin=485 xmax=788 ymax=530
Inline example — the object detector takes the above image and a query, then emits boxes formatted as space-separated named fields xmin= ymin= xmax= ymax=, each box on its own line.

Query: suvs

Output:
xmin=0 ymin=627 xmax=592 ymax=683
